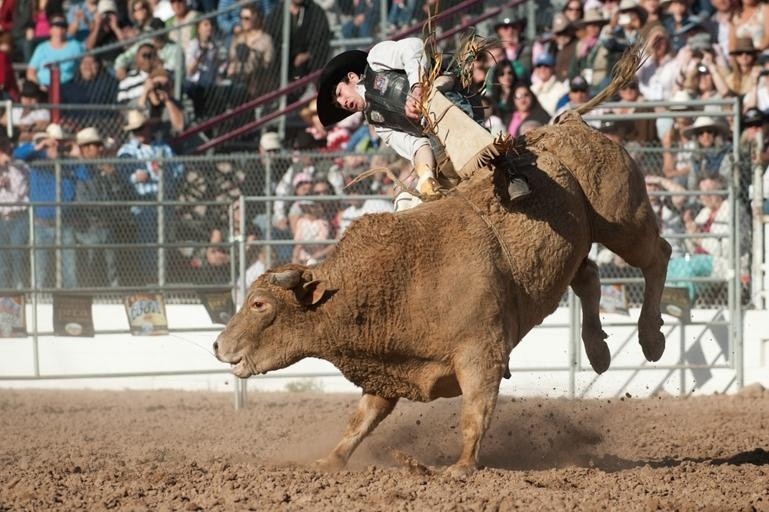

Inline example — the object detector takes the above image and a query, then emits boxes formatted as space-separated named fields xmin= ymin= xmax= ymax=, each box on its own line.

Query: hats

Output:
xmin=260 ymin=132 xmax=282 ymax=151
xmin=494 ymin=1 xmax=769 ymax=139
xmin=75 ymin=127 xmax=103 ymax=146
xmin=317 ymin=50 xmax=367 ymax=127
xmin=123 ymin=110 xmax=159 ymax=132
xmin=293 ymin=172 xmax=313 ymax=188
xmin=97 ymin=1 xmax=118 ymax=16
xmin=50 ymin=15 xmax=70 ymax=29
xmin=20 ymin=82 xmax=40 ymax=96
xmin=298 ymin=99 xmax=317 ymax=126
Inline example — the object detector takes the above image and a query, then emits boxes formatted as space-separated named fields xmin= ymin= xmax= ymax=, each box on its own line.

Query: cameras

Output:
xmin=697 ymin=65 xmax=708 ymax=74
xmin=154 ymin=83 xmax=161 ymax=90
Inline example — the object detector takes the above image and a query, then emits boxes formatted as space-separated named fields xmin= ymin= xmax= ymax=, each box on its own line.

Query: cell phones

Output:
xmin=58 ymin=145 xmax=71 ymax=152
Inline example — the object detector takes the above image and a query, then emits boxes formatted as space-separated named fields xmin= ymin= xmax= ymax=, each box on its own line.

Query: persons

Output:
xmin=1 ymin=0 xmax=769 ymax=307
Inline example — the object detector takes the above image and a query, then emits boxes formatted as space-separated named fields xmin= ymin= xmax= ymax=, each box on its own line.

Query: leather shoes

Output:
xmin=506 ymin=177 xmax=530 ymax=201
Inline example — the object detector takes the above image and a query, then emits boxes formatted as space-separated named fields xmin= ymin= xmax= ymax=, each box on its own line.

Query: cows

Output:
xmin=211 ymin=35 xmax=672 ymax=481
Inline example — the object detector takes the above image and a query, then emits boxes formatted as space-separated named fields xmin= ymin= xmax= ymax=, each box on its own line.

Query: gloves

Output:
xmin=415 ymin=163 xmax=442 ymax=200
